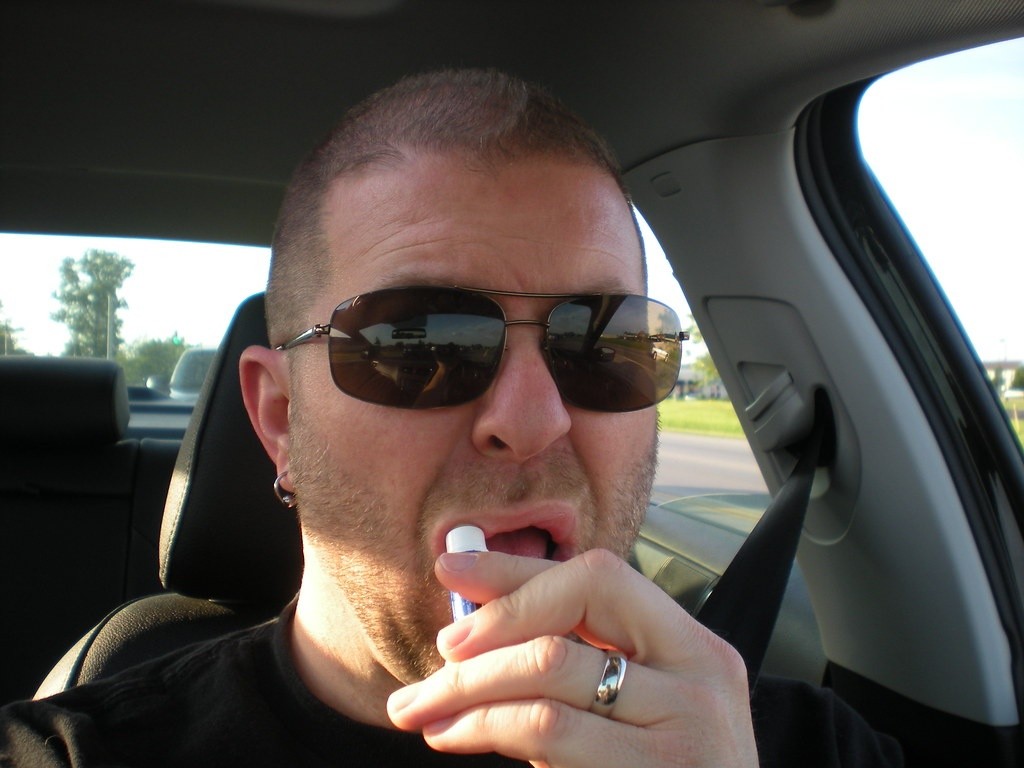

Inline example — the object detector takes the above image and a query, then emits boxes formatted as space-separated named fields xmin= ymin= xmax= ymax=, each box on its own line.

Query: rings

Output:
xmin=589 ymin=650 xmax=626 ymax=719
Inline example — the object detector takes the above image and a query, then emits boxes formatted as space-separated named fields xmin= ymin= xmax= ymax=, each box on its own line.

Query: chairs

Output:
xmin=1 ymin=294 xmax=305 ymax=701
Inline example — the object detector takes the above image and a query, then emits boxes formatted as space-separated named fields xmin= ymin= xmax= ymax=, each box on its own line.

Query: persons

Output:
xmin=1 ymin=69 xmax=911 ymax=765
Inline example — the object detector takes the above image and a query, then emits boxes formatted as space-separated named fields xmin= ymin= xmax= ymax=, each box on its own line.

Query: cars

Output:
xmin=142 ymin=347 xmax=217 ymax=409
xmin=650 ymin=346 xmax=670 ymax=363
xmin=126 ymin=384 xmax=193 ymax=440
xmin=621 ymin=329 xmax=680 ymax=343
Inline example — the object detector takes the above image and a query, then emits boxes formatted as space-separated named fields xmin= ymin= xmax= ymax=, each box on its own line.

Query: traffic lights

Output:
xmin=173 ymin=333 xmax=184 ymax=345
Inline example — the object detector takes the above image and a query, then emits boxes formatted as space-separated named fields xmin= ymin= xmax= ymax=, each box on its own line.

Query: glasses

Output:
xmin=273 ymin=284 xmax=689 ymax=413
